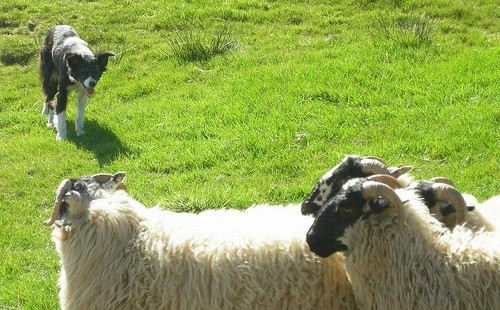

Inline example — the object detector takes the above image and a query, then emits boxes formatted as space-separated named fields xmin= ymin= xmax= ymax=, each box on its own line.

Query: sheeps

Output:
xmin=300 ymin=153 xmax=500 ymax=310
xmin=42 ymin=170 xmax=358 ymax=310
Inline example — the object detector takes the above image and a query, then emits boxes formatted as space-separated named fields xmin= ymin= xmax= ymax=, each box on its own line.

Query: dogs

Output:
xmin=38 ymin=24 xmax=116 ymax=144
